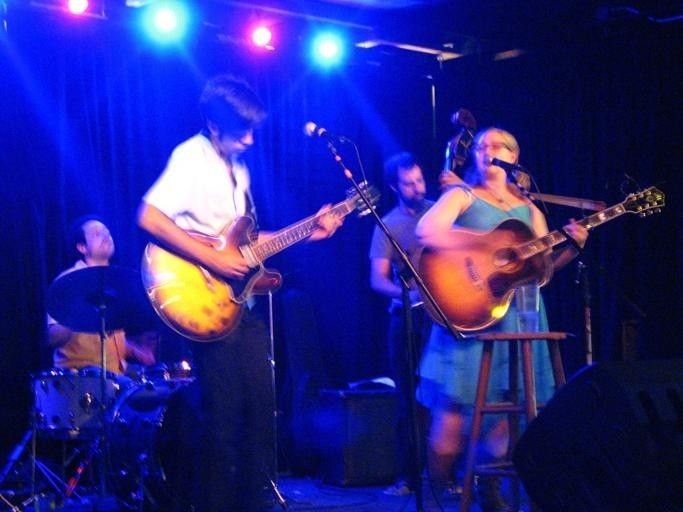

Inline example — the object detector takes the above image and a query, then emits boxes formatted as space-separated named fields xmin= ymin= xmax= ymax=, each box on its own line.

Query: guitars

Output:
xmin=412 ymin=183 xmax=666 ymax=334
xmin=140 ymin=181 xmax=380 ymax=347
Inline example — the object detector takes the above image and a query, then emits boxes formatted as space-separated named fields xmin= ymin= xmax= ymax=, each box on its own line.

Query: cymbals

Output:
xmin=43 ymin=266 xmax=145 ymax=336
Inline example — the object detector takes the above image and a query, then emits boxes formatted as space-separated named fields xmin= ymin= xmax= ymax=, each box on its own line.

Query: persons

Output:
xmin=414 ymin=126 xmax=589 ymax=512
xmin=366 ymin=151 xmax=466 ymax=499
xmin=46 ymin=212 xmax=154 ymax=376
xmin=137 ymin=73 xmax=343 ymax=511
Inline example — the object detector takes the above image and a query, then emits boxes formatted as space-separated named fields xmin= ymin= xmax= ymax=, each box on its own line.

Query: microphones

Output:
xmin=484 ymin=154 xmax=529 ymax=175
xmin=303 ymin=121 xmax=345 ymax=142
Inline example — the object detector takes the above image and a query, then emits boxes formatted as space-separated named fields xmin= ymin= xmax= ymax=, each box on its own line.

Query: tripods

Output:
xmin=54 ymin=299 xmax=160 ymax=512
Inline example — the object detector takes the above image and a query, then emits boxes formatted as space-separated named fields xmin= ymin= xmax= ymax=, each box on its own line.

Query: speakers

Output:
xmin=316 ymin=386 xmax=406 ymax=487
xmin=510 ymin=361 xmax=683 ymax=511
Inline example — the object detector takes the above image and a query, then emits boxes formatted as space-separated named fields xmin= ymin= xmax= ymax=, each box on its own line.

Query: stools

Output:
xmin=460 ymin=329 xmax=568 ymax=506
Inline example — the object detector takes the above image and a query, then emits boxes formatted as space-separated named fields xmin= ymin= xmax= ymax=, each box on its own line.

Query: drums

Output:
xmin=97 ymin=375 xmax=194 ymax=512
xmin=123 ymin=363 xmax=192 ymax=384
xmin=29 ymin=369 xmax=117 ymax=441
